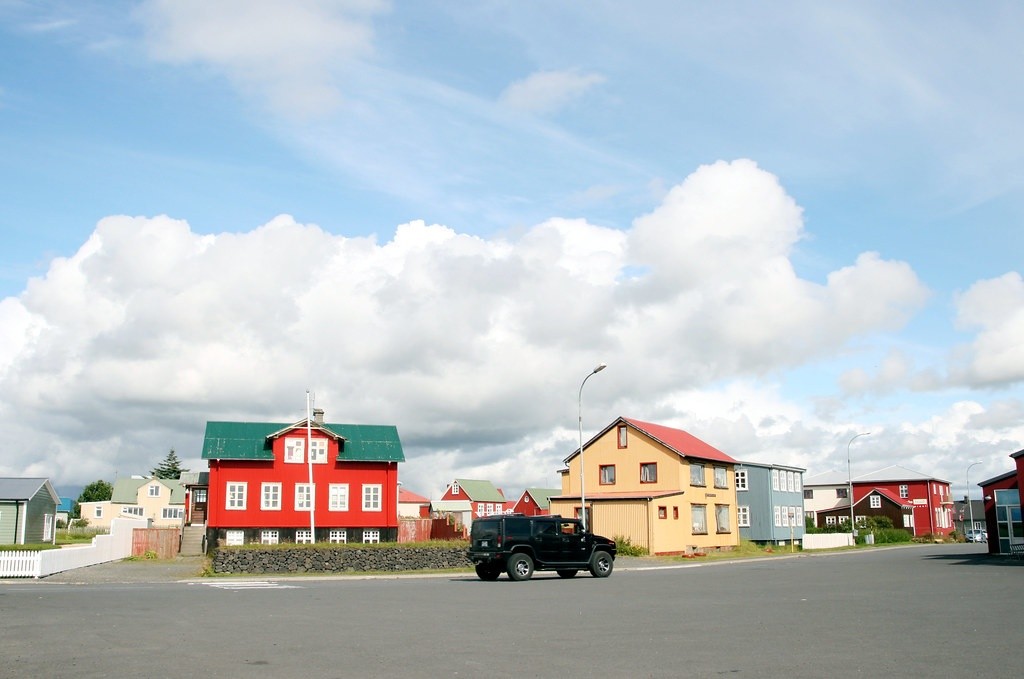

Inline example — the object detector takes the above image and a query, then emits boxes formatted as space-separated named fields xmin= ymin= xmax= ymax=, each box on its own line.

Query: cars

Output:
xmin=963 ymin=529 xmax=988 ymax=543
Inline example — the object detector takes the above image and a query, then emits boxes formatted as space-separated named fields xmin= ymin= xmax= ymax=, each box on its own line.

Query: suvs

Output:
xmin=464 ymin=513 xmax=618 ymax=581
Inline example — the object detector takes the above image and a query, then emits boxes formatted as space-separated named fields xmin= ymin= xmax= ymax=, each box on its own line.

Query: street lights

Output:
xmin=577 ymin=363 xmax=606 ymax=530
xmin=965 ymin=461 xmax=982 ymax=544
xmin=846 ymin=432 xmax=871 ymax=546
